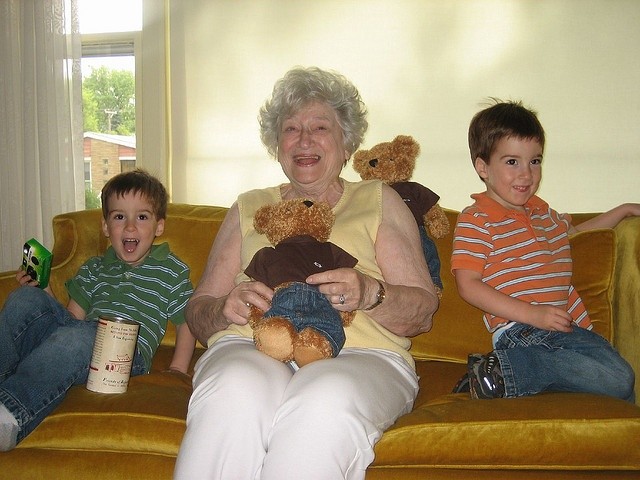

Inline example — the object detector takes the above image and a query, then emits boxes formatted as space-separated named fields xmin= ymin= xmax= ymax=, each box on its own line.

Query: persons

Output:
xmin=174 ymin=65 xmax=440 ymax=474
xmin=0 ymin=169 xmax=196 ymax=452
xmin=450 ymin=96 xmax=635 ymax=400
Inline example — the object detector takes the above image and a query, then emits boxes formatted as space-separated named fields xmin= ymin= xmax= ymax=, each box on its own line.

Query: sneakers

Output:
xmin=454 ymin=372 xmax=469 ymax=392
xmin=468 ymin=354 xmax=504 ymax=400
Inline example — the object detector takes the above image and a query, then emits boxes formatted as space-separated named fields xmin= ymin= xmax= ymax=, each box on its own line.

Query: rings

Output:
xmin=340 ymin=295 xmax=345 ymax=304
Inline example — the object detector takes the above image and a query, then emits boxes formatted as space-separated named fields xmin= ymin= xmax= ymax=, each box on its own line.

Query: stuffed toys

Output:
xmin=244 ymin=194 xmax=358 ymax=367
xmin=352 ymin=135 xmax=451 ymax=300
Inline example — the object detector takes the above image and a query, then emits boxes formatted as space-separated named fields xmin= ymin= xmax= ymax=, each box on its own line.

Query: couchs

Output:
xmin=0 ymin=203 xmax=639 ymax=480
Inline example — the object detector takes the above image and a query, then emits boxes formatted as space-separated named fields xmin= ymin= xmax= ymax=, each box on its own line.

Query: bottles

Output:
xmin=85 ymin=315 xmax=140 ymax=396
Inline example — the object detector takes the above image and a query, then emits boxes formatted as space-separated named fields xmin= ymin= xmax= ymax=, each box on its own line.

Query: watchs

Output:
xmin=365 ymin=279 xmax=386 ymax=311
